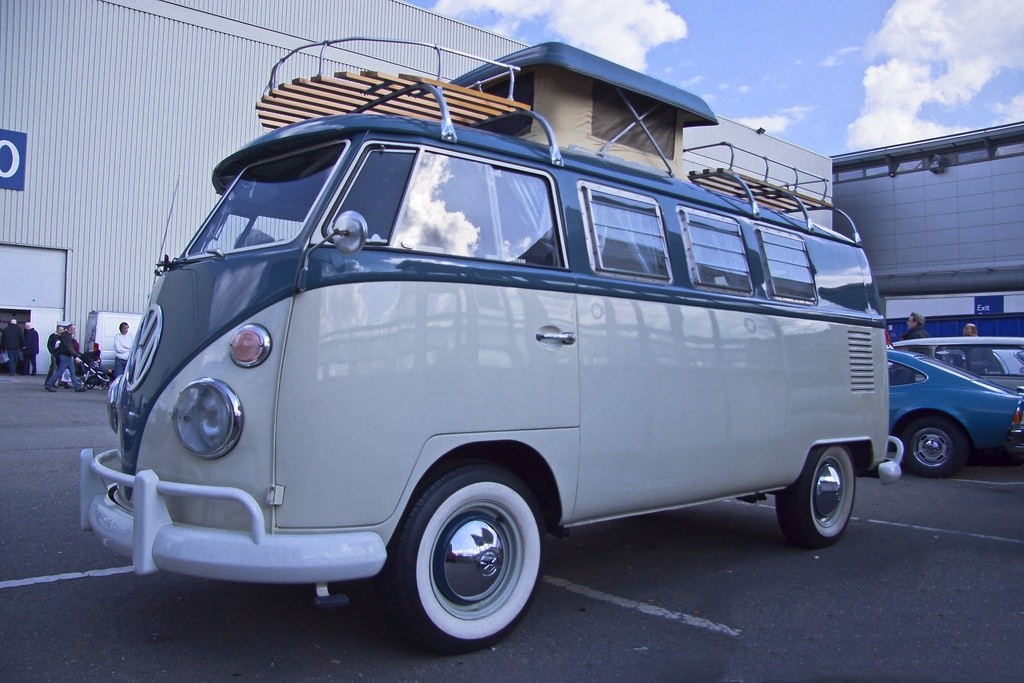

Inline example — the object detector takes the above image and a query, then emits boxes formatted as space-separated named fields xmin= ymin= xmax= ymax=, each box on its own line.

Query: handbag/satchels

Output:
xmin=0 ymin=351 xmax=9 ymax=364
xmin=73 ymin=339 xmax=79 ymax=353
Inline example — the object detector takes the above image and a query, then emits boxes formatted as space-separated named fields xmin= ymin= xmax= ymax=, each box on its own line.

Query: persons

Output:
xmin=964 ymin=323 xmax=995 ymax=375
xmin=1 ymin=318 xmax=26 ymax=376
xmin=114 ymin=322 xmax=134 ymax=381
xmin=906 ymin=311 xmax=932 ymax=353
xmin=22 ymin=321 xmax=39 ymax=375
xmin=92 ymin=358 xmax=101 ymax=367
xmin=44 ymin=324 xmax=86 ymax=392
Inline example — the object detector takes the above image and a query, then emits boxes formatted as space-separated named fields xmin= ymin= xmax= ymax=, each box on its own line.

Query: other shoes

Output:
xmin=44 ymin=380 xmax=87 ymax=392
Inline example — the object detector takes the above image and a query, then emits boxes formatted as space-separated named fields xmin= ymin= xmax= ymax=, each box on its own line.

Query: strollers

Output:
xmin=81 ymin=350 xmax=111 ymax=389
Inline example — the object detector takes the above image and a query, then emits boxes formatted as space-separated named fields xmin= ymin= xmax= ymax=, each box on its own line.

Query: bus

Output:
xmin=77 ymin=83 xmax=905 ymax=655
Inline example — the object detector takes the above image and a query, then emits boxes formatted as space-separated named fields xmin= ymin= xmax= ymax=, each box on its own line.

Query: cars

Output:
xmin=888 ymin=350 xmax=1024 ymax=478
xmin=892 ymin=337 xmax=1024 ymax=393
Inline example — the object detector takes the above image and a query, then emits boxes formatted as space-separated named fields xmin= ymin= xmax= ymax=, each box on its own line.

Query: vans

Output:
xmin=84 ymin=310 xmax=142 ymax=369
